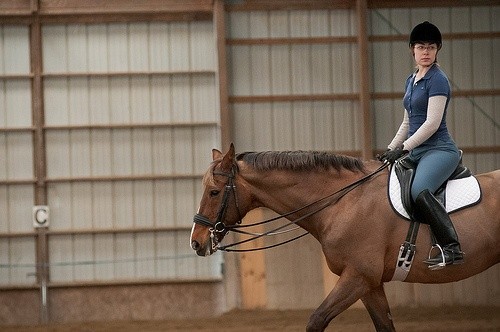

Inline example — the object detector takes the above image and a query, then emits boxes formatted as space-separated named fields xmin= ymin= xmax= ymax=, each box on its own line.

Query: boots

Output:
xmin=415 ymin=189 xmax=464 ymax=265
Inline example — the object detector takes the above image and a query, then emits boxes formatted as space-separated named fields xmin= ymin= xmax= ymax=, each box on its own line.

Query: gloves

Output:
xmin=378 ymin=149 xmax=391 ymax=162
xmin=382 ymin=144 xmax=409 ymax=164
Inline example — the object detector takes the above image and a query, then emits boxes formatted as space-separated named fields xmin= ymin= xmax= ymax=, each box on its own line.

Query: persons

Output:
xmin=375 ymin=21 xmax=465 ymax=264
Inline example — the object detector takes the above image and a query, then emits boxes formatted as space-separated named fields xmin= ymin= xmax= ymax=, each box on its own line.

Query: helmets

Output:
xmin=409 ymin=21 xmax=442 ymax=51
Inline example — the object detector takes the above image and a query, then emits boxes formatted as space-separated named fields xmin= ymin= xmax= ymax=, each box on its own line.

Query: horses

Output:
xmin=190 ymin=143 xmax=500 ymax=332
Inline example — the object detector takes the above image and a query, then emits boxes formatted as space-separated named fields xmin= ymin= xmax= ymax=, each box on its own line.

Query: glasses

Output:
xmin=413 ymin=45 xmax=438 ymax=51
